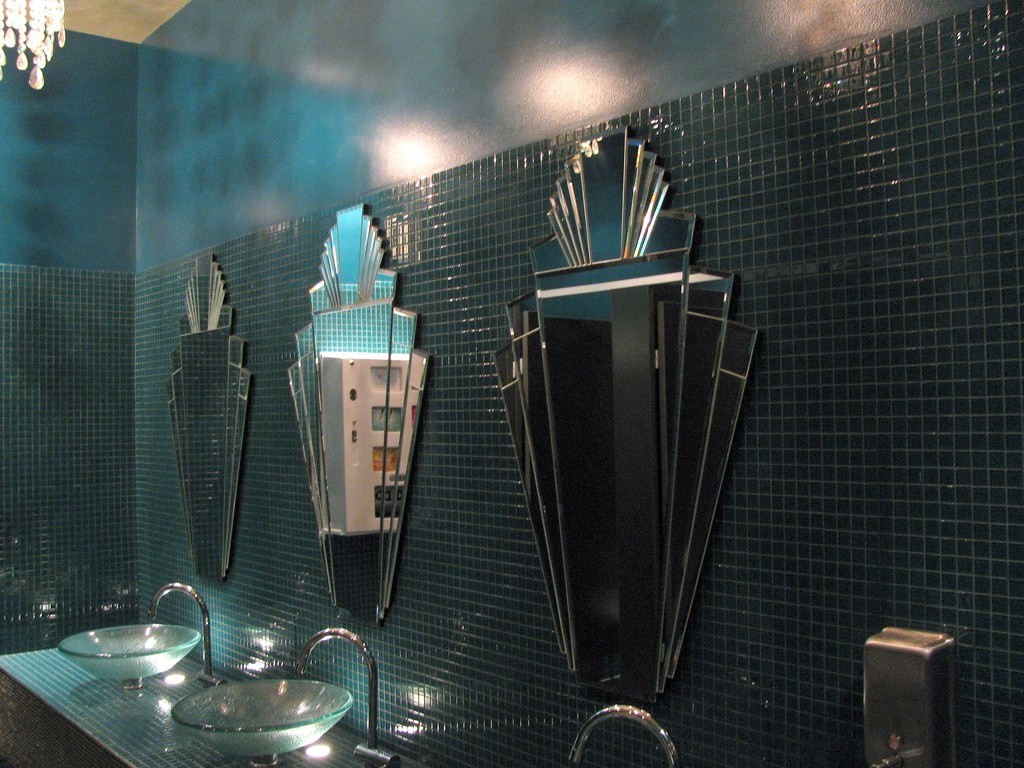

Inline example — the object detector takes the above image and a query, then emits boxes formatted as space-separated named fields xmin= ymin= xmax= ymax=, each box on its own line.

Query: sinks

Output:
xmin=170 ymin=678 xmax=354 ymax=759
xmin=57 ymin=622 xmax=201 ymax=680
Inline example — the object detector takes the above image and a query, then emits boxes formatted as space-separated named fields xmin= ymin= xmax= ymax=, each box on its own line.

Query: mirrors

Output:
xmin=285 ymin=201 xmax=433 ymax=627
xmin=167 ymin=252 xmax=253 ymax=582
xmin=493 ymin=125 xmax=759 ymax=701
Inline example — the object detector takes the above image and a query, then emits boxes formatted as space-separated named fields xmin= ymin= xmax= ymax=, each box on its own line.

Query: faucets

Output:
xmin=294 ymin=627 xmax=403 ymax=768
xmin=148 ymin=582 xmax=228 ymax=689
xmin=567 ymin=704 xmax=681 ymax=768
